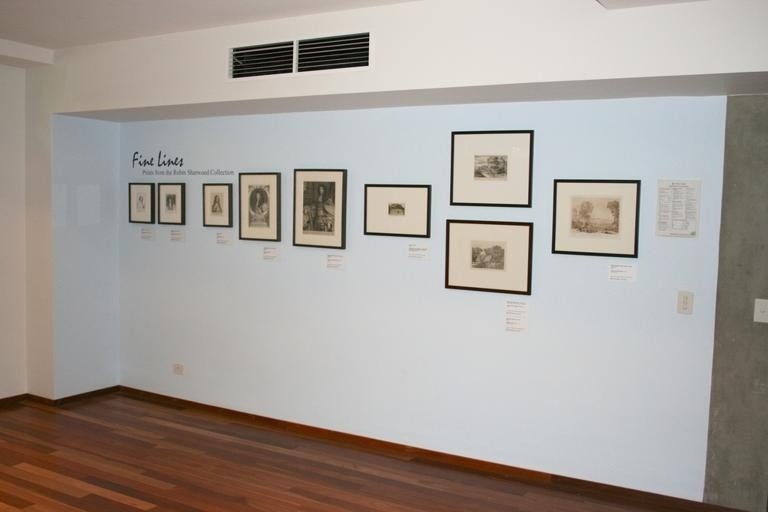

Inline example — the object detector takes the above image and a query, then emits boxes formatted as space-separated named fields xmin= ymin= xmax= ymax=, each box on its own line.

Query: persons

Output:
xmin=211 ymin=196 xmax=223 ymax=213
xmin=310 ymin=184 xmax=334 ymax=232
xmin=137 ymin=196 xmax=145 ymax=208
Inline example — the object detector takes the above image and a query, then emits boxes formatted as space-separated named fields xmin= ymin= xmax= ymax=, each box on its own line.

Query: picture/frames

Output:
xmin=129 ymin=183 xmax=185 ymax=227
xmin=552 ymin=179 xmax=641 ymax=260
xmin=203 ymin=169 xmax=347 ymax=249
xmin=444 ymin=130 xmax=535 ymax=296
xmin=364 ymin=184 xmax=432 ymax=239
